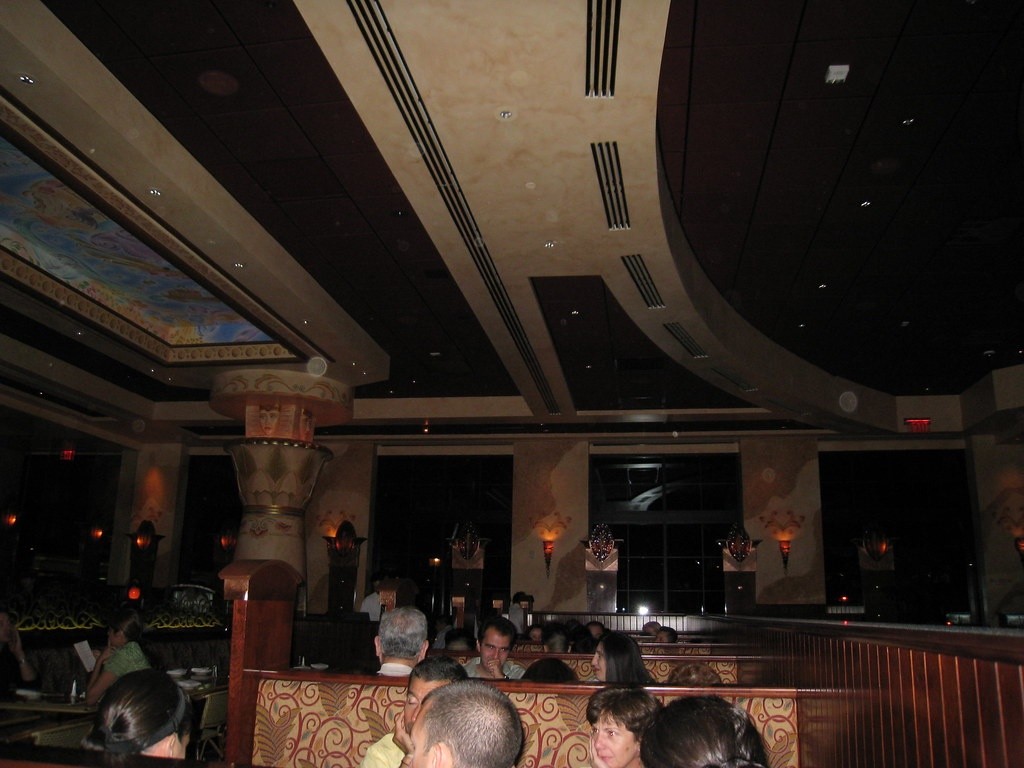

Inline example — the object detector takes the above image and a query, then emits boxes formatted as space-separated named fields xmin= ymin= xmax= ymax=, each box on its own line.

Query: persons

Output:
xmin=528 ymin=619 xmax=606 ymax=654
xmin=509 ymin=591 xmax=527 ymax=633
xmin=521 ymin=658 xmax=576 ymax=680
xmin=464 ymin=617 xmax=526 ymax=680
xmin=585 ymin=683 xmax=662 ymax=767
xmin=592 ymin=632 xmax=654 ymax=682
xmin=432 ymin=616 xmax=474 ymax=662
xmin=669 ymin=661 xmax=722 ymax=684
xmin=79 ymin=669 xmax=192 ymax=768
xmin=374 ymin=607 xmax=429 ymax=676
xmin=408 ymin=681 xmax=523 ymax=768
xmin=359 ymin=657 xmax=468 ymax=768
xmin=69 ymin=613 xmax=153 ymax=705
xmin=640 ymin=697 xmax=770 ymax=768
xmin=642 ymin=621 xmax=678 ymax=642
xmin=0 ymin=608 xmax=39 ymax=702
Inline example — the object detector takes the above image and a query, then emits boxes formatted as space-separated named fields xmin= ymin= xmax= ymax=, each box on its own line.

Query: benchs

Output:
xmin=7 ymin=626 xmax=229 ymax=691
xmin=256 ymin=629 xmax=800 ymax=768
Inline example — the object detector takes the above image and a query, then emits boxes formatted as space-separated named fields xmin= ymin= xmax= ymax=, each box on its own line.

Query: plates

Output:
xmin=312 ymin=663 xmax=328 ymax=671
xmin=167 ymin=669 xmax=187 ymax=679
xmin=176 ymin=680 xmax=201 ymax=690
xmin=190 ymin=667 xmax=213 ymax=676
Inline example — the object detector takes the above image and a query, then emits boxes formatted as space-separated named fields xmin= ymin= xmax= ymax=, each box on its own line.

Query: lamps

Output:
xmin=323 ymin=535 xmax=335 ymax=563
xmin=778 ymin=540 xmax=790 ymax=567
xmin=540 ymin=540 xmax=554 ymax=578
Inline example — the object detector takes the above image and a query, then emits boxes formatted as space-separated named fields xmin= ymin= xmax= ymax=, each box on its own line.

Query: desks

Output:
xmin=0 ymin=674 xmax=229 ymax=714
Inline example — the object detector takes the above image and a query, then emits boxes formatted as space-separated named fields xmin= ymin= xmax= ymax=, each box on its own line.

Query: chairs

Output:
xmin=31 ymin=719 xmax=95 ymax=749
xmin=190 ymin=689 xmax=228 ymax=760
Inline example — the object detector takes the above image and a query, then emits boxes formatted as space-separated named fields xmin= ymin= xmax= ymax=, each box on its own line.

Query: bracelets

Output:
xmin=18 ymin=658 xmax=27 ymax=664
xmin=505 ymin=675 xmax=509 ymax=680
xmin=402 ymin=760 xmax=408 ymax=766
